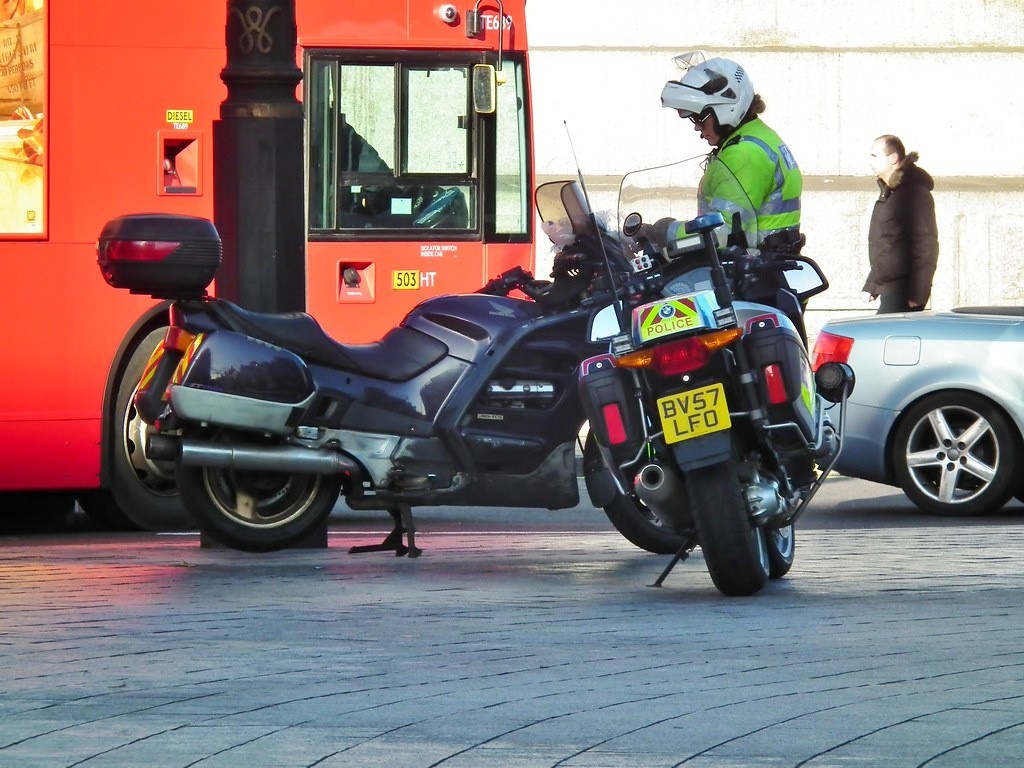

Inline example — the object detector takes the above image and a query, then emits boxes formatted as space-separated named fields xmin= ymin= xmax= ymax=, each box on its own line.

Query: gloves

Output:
xmin=649 ymin=217 xmax=675 ymax=247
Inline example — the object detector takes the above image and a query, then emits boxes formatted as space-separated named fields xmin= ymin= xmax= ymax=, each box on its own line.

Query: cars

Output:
xmin=810 ymin=306 xmax=1024 ymax=515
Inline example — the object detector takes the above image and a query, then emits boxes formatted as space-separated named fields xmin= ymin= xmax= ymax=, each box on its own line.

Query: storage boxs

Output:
xmin=96 ymin=212 xmax=224 ymax=293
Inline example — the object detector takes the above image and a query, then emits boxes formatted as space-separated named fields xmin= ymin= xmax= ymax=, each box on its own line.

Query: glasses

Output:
xmin=688 ymin=110 xmax=713 ymax=127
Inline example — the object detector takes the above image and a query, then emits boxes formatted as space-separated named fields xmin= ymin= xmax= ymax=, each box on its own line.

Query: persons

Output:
xmin=658 ymin=50 xmax=805 ymax=253
xmin=862 ymin=135 xmax=940 ymax=315
xmin=318 ymin=100 xmax=397 ymax=225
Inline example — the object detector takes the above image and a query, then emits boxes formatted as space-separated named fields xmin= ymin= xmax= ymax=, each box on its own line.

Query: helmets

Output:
xmin=661 ymin=57 xmax=755 ymax=128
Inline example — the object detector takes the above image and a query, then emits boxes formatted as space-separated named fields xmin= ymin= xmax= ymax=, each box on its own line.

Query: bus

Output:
xmin=2 ymin=1 xmax=536 ymax=532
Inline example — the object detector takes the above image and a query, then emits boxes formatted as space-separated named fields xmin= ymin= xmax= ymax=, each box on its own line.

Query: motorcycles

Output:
xmin=95 ymin=118 xmax=698 ymax=561
xmin=578 ymin=153 xmax=857 ymax=596
